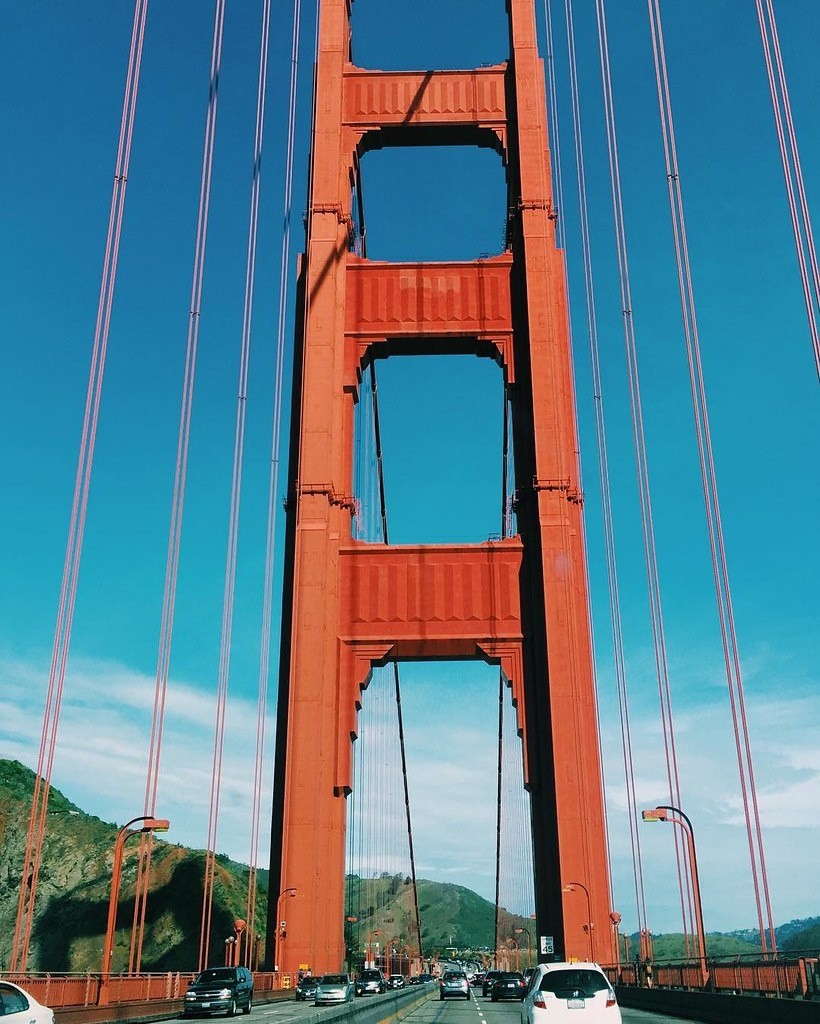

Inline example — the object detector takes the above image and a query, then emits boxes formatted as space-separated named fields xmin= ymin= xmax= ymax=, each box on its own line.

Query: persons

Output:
xmin=633 ymin=954 xmax=644 ymax=988
xmin=642 ymin=955 xmax=653 ymax=989
xmin=298 ymin=969 xmax=304 ymax=983
xmin=307 ymin=969 xmax=312 ymax=978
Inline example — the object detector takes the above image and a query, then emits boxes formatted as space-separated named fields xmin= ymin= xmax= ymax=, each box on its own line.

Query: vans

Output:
xmin=420 ymin=974 xmax=433 ymax=984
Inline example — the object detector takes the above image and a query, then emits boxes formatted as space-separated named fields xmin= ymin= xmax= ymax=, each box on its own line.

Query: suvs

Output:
xmin=183 ymin=966 xmax=255 ymax=1018
xmin=354 ymin=969 xmax=386 ymax=997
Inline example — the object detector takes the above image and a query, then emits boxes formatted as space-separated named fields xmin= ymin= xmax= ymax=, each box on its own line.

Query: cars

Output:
xmin=409 ymin=977 xmax=421 ymax=985
xmin=444 ymin=958 xmax=472 ymax=970
xmin=438 ymin=971 xmax=471 ymax=1001
xmin=473 ymin=967 xmax=534 ymax=1002
xmin=387 ymin=974 xmax=405 ymax=989
xmin=295 ymin=977 xmax=322 ymax=1001
xmin=314 ymin=973 xmax=355 ymax=1006
xmin=519 ymin=961 xmax=622 ymax=1024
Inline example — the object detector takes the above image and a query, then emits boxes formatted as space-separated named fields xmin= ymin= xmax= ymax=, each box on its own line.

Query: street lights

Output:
xmin=98 ymin=816 xmax=170 ymax=1006
xmin=273 ymin=887 xmax=297 ymax=989
xmin=643 ymin=806 xmax=710 ymax=987
xmin=515 ymin=928 xmax=533 ymax=965
xmin=564 ymin=882 xmax=597 ymax=965
xmin=608 ymin=912 xmax=621 ymax=983
xmin=500 ymin=945 xmax=511 ymax=971
xmin=507 ymin=938 xmax=519 ymax=970
